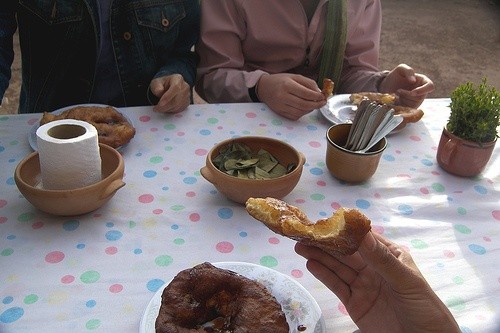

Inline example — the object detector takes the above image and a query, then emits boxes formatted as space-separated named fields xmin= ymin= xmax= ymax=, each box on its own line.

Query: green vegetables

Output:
xmin=447 ymin=77 xmax=500 ymax=145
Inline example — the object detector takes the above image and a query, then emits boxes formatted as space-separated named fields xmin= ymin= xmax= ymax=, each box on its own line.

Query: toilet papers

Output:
xmin=36 ymin=119 xmax=102 ymax=191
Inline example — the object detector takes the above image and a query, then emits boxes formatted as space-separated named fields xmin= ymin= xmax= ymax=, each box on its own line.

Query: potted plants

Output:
xmin=436 ymin=77 xmax=500 ymax=177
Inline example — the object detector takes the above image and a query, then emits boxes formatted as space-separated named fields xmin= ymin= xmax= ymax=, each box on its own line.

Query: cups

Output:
xmin=327 ymin=123 xmax=387 ymax=184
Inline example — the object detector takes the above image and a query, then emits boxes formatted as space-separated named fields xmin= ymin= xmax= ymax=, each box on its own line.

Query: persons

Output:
xmin=193 ymin=0 xmax=434 ymax=121
xmin=0 ymin=0 xmax=201 ymax=114
xmin=295 ymin=218 xmax=463 ymax=333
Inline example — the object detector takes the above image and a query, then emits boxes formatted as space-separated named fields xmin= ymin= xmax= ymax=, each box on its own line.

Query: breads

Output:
xmin=155 ymin=261 xmax=290 ymax=333
xmin=320 ymin=78 xmax=335 ymax=99
xmin=39 ymin=105 xmax=136 ymax=147
xmin=245 ymin=196 xmax=372 ymax=257
xmin=348 ymin=86 xmax=424 ymax=131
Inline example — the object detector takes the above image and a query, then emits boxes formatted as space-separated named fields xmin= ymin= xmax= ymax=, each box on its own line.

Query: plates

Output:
xmin=319 ymin=94 xmax=404 ymax=135
xmin=27 ymin=104 xmax=135 ymax=151
xmin=139 ymin=261 xmax=325 ymax=333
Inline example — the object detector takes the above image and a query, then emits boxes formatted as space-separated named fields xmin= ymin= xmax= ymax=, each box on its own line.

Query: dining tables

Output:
xmin=0 ymin=96 xmax=500 ymax=333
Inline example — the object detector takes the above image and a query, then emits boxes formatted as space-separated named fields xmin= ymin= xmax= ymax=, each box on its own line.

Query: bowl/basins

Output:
xmin=15 ymin=143 xmax=125 ymax=217
xmin=199 ymin=135 xmax=305 ymax=205
